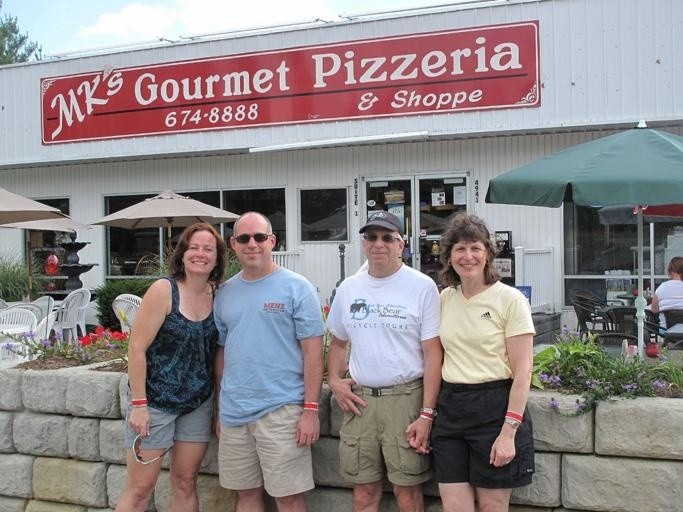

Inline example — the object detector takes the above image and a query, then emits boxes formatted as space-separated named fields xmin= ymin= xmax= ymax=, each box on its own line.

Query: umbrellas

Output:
xmin=485 ymin=120 xmax=683 ymax=361
xmin=0 ymin=186 xmax=70 ymax=227
xmin=597 ymin=202 xmax=683 ymax=296
xmin=0 ymin=215 xmax=94 ymax=299
xmin=86 ymin=188 xmax=241 ymax=267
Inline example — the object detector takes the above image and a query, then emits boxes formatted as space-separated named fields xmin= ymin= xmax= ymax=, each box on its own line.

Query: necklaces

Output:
xmin=183 ymin=283 xmax=208 ymax=328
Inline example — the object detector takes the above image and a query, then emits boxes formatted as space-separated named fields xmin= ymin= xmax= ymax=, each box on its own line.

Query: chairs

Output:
xmin=0 ymin=288 xmax=91 ymax=369
xmin=569 ymin=286 xmax=683 ymax=349
xmin=112 ymin=294 xmax=143 ymax=337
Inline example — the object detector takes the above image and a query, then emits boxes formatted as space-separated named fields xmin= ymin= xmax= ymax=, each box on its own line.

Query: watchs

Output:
xmin=505 ymin=418 xmax=520 ymax=430
xmin=419 ymin=406 xmax=437 ymax=417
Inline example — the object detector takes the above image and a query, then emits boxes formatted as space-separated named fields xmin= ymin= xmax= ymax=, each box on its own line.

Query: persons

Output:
xmin=112 ymin=223 xmax=228 ymax=511
xmin=404 ymin=211 xmax=536 ymax=512
xmin=325 ymin=210 xmax=443 ymax=511
xmin=213 ymin=212 xmax=325 ymax=511
xmin=651 ymin=256 xmax=683 ymax=350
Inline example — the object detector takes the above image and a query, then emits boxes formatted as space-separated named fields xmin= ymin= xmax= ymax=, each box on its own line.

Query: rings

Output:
xmin=312 ymin=437 xmax=316 ymax=441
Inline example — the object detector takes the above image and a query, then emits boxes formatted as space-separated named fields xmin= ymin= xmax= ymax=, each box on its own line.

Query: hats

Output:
xmin=359 ymin=211 xmax=402 ymax=233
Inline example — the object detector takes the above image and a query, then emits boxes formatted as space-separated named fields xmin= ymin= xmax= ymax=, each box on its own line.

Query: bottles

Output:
xmin=644 ymin=287 xmax=652 ymax=305
xmin=621 ymin=339 xmax=637 ymax=365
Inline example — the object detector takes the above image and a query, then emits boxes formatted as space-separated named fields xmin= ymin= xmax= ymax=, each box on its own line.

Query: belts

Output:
xmin=362 ymin=377 xmax=423 ymax=396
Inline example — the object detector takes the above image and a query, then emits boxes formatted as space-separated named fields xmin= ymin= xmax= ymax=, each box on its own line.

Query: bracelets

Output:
xmin=301 ymin=401 xmax=318 ymax=411
xmin=420 ymin=412 xmax=435 ymax=420
xmin=129 ymin=398 xmax=147 ymax=406
xmin=505 ymin=410 xmax=523 ymax=422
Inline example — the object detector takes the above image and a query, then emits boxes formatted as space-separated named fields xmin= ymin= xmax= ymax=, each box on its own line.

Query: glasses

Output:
xmin=363 ymin=235 xmax=402 ymax=242
xmin=234 ymin=232 xmax=272 ymax=243
xmin=132 ymin=423 xmax=175 ymax=465
